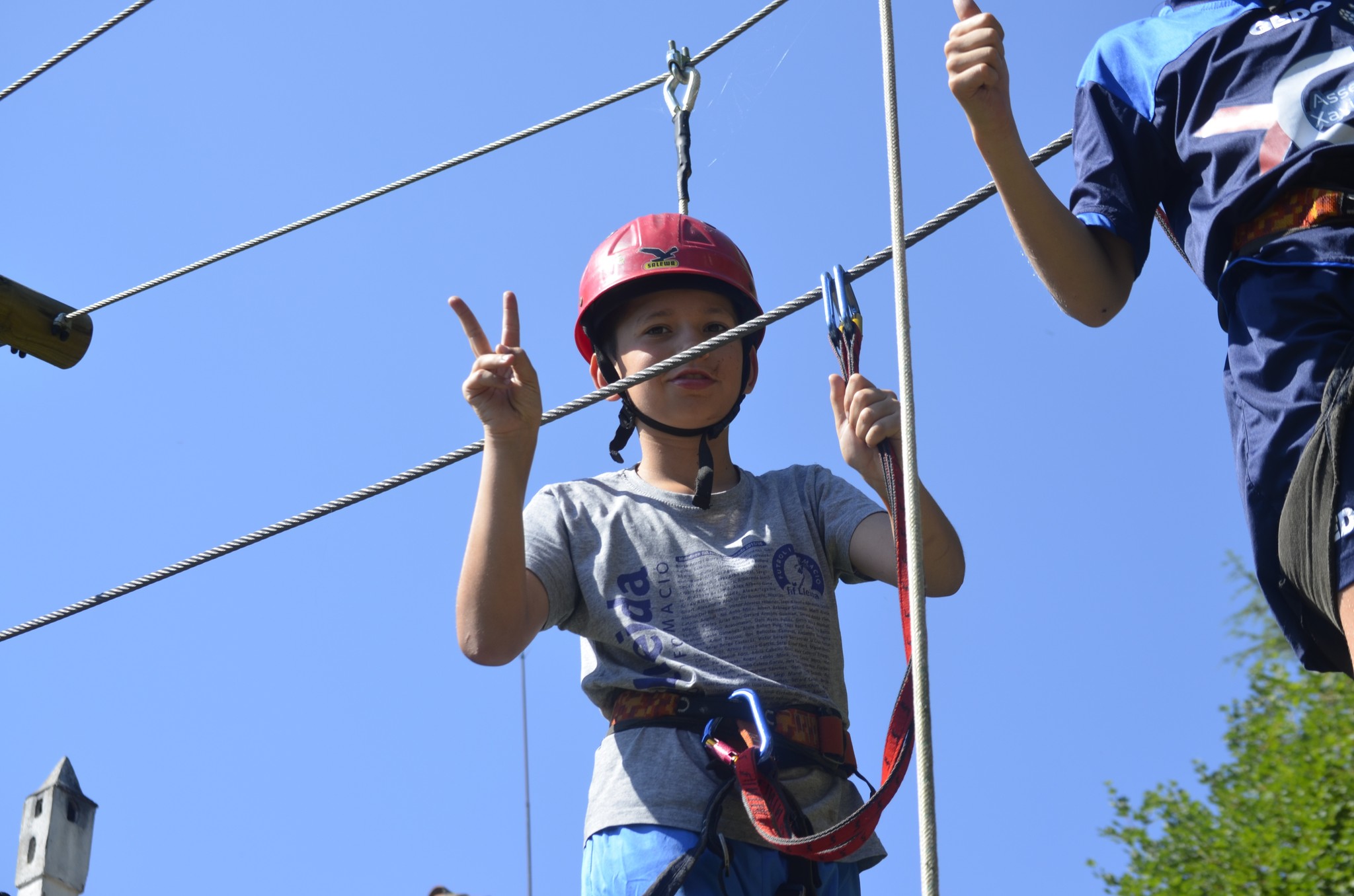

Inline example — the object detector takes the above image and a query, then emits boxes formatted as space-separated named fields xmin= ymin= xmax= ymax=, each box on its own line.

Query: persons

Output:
xmin=943 ymin=0 xmax=1353 ymax=680
xmin=449 ymin=214 xmax=968 ymax=896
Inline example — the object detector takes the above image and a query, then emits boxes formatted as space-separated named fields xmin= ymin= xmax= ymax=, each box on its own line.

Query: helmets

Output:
xmin=573 ymin=213 xmax=767 ymax=365
